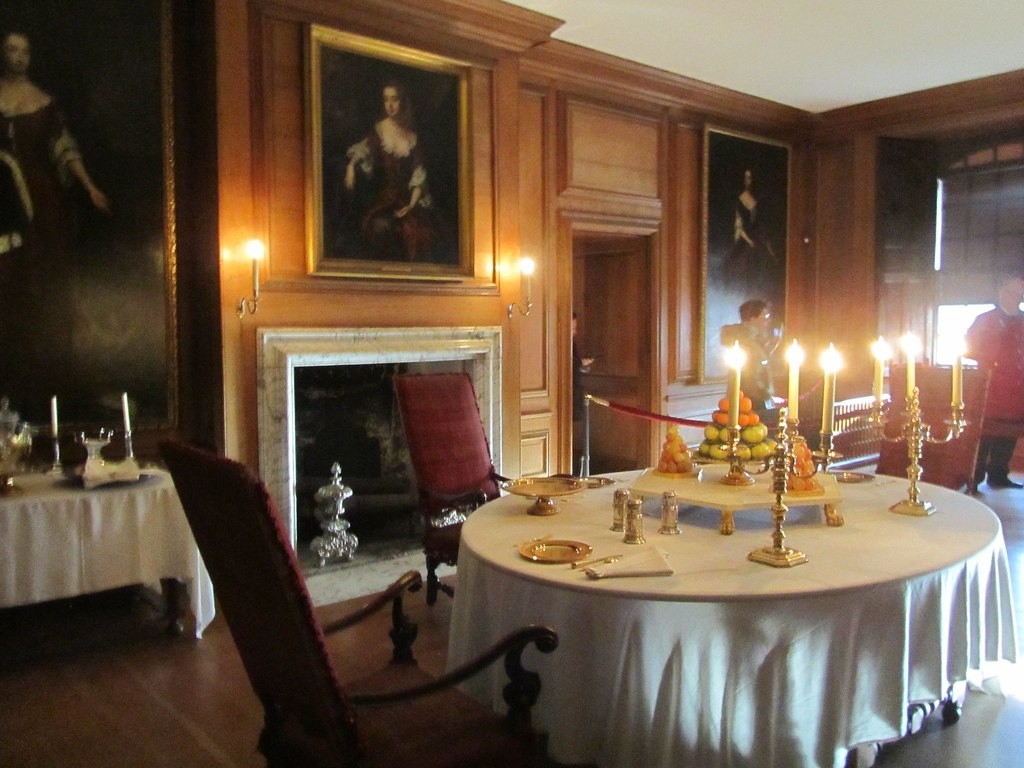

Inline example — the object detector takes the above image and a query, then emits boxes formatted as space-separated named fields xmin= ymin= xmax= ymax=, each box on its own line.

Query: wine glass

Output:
xmin=74 ymin=427 xmax=114 ymax=465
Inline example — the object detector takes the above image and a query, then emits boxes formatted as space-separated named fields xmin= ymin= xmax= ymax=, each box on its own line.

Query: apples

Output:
xmin=699 ymin=425 xmax=778 ymax=460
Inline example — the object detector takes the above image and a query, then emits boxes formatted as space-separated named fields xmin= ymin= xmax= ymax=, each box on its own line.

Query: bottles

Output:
xmin=611 ymin=488 xmax=630 ymax=531
xmin=624 ymin=498 xmax=645 ymax=544
xmin=659 ymin=492 xmax=682 ymax=534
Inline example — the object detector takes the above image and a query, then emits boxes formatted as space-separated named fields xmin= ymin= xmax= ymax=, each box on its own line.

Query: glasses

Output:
xmin=758 ymin=314 xmax=770 ymax=319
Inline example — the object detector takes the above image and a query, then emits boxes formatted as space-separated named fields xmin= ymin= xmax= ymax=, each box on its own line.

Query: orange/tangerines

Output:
xmin=712 ymin=389 xmax=758 ymax=426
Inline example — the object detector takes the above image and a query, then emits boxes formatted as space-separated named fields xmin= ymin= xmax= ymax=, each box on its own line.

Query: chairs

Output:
xmin=158 ymin=426 xmax=565 ymax=768
xmin=394 ymin=370 xmax=514 ymax=609
xmin=867 ymin=355 xmax=997 ymax=503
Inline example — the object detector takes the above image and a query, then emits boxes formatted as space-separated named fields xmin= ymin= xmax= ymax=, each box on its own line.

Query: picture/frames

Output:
xmin=698 ymin=120 xmax=796 ymax=389
xmin=301 ymin=17 xmax=481 ymax=284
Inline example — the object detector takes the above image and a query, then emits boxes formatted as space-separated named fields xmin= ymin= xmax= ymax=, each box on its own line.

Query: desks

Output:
xmin=0 ymin=464 xmax=217 ymax=650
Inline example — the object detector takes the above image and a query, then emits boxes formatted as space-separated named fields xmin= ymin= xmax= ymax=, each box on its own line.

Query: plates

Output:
xmin=521 ymin=539 xmax=592 ymax=562
xmin=828 ymin=472 xmax=875 ymax=482
xmin=68 ymin=474 xmax=151 ymax=487
xmin=572 ymin=477 xmax=613 ymax=487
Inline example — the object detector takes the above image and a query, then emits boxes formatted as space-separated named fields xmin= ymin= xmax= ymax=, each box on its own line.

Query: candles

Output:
xmin=120 ymin=389 xmax=132 ymax=433
xmin=782 ymin=337 xmax=807 ymax=417
xmin=951 ymin=348 xmax=965 ymax=405
xmin=871 ymin=338 xmax=887 ymax=401
xmin=819 ymin=342 xmax=841 ymax=431
xmin=902 ymin=333 xmax=922 ymax=396
xmin=51 ymin=392 xmax=60 ymax=436
xmin=726 ymin=341 xmax=743 ymax=427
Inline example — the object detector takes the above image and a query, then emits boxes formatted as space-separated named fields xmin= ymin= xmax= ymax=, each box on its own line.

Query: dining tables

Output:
xmin=446 ymin=466 xmax=1018 ymax=768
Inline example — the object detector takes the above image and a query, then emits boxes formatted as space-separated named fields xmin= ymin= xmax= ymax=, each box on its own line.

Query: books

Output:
xmin=585 ymin=547 xmax=674 ymax=579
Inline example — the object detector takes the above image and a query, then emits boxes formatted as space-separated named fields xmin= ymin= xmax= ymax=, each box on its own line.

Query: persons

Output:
xmin=720 ymin=300 xmax=784 ymax=424
xmin=573 ymin=310 xmax=595 ymax=475
xmin=964 ymin=264 xmax=1024 ymax=496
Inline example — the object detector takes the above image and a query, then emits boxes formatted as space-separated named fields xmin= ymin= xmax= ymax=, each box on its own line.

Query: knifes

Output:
xmin=574 ymin=554 xmax=623 ymax=567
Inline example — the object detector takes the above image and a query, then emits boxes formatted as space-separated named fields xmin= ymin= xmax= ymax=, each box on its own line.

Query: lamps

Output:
xmin=504 ymin=256 xmax=537 ymax=321
xmin=233 ymin=239 xmax=272 ymax=320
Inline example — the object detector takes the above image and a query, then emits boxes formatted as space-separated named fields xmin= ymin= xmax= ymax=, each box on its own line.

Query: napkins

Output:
xmin=583 ymin=539 xmax=674 ymax=578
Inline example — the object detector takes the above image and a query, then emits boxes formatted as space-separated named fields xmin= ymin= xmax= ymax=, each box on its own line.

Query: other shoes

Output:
xmin=987 ymin=475 xmax=1023 ymax=488
